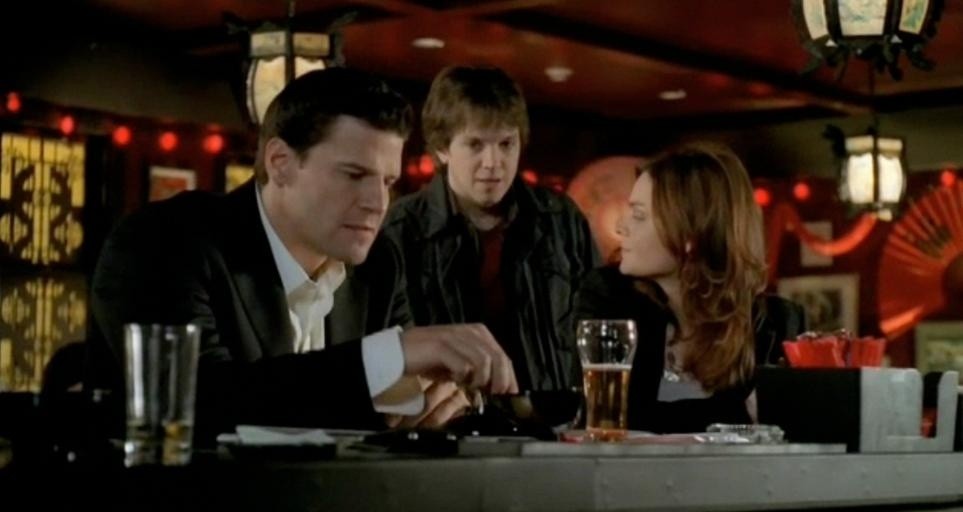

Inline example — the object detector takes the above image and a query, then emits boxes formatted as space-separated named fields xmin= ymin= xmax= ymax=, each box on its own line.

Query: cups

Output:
xmin=575 ymin=319 xmax=639 ymax=440
xmin=120 ymin=322 xmax=201 ymax=458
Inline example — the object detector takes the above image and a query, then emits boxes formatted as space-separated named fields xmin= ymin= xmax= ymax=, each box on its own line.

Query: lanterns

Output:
xmin=837 ymin=133 xmax=906 ymax=221
xmin=245 ymin=16 xmax=330 ymax=127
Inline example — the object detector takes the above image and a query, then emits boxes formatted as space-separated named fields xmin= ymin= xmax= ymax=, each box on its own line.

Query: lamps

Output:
xmin=836 ymin=69 xmax=908 ymax=224
xmin=242 ymin=4 xmax=344 ymax=140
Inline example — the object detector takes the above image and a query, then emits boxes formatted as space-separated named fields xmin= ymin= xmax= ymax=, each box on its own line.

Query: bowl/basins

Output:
xmin=491 ymin=390 xmax=577 ymax=439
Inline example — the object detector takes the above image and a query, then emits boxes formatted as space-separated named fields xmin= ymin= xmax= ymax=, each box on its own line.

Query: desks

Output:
xmin=94 ymin=406 xmax=963 ymax=506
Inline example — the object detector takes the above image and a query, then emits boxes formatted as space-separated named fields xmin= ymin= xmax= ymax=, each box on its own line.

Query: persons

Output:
xmin=91 ymin=67 xmax=520 ymax=449
xmin=574 ymin=141 xmax=818 ymax=444
xmin=363 ymin=64 xmax=608 ymax=437
